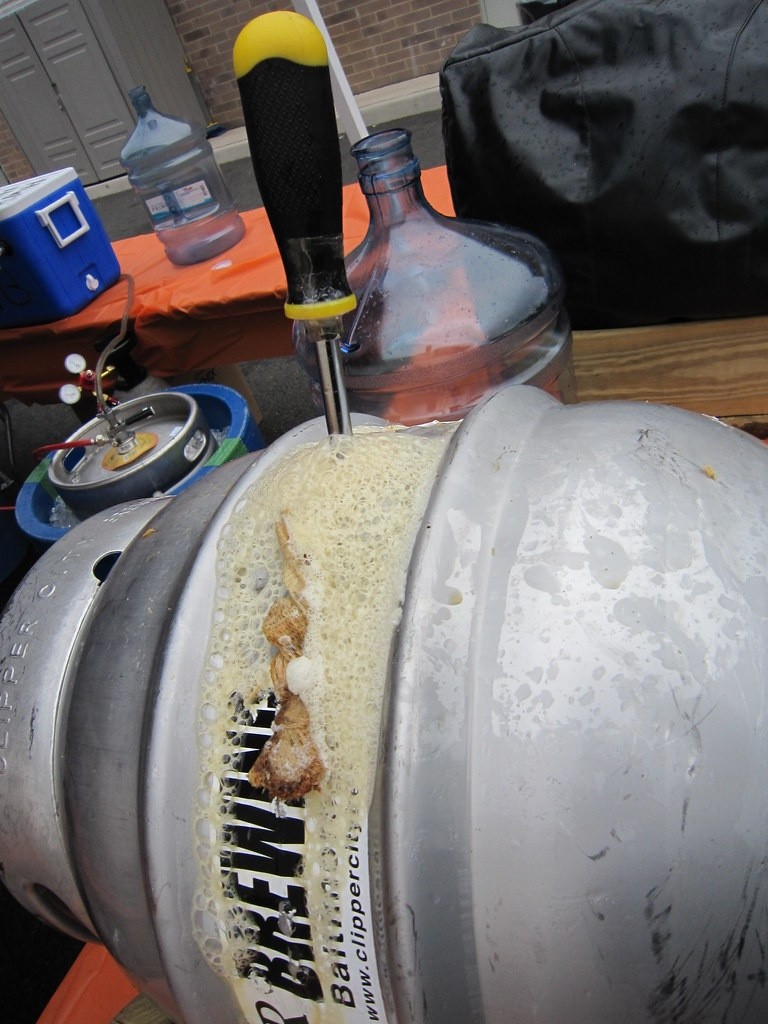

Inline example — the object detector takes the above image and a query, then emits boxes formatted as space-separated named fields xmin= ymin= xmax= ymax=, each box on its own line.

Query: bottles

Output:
xmin=118 ymin=87 xmax=245 ymax=265
xmin=292 ymin=126 xmax=574 ymax=426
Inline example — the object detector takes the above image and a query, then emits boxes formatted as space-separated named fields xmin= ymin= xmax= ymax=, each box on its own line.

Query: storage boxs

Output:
xmin=0 ymin=167 xmax=122 ymax=328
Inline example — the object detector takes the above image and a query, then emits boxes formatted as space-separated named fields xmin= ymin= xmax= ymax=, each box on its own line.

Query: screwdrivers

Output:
xmin=228 ymin=8 xmax=365 ymax=443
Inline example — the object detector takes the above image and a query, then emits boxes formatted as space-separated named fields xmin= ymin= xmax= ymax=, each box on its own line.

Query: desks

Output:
xmin=0 ymin=165 xmax=459 ymax=395
